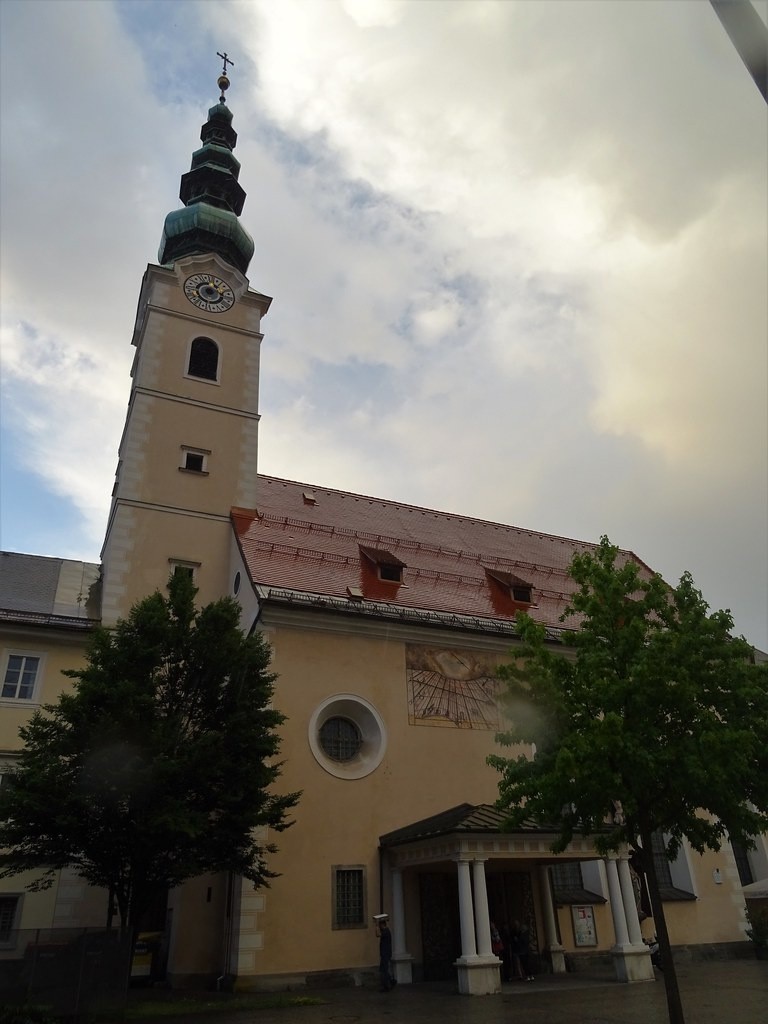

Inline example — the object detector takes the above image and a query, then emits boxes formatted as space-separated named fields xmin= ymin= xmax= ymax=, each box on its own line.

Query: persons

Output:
xmin=376 ymin=919 xmax=393 ymax=984
xmin=490 ymin=917 xmax=535 ymax=983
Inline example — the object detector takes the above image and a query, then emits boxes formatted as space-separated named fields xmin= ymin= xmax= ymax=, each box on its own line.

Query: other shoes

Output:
xmin=378 ymin=988 xmax=392 ymax=993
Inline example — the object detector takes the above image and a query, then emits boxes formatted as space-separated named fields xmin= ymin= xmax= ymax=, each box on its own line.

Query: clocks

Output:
xmin=183 ymin=273 xmax=235 ymax=314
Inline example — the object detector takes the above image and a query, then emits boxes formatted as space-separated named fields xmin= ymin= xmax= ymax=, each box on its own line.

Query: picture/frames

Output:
xmin=571 ymin=903 xmax=599 ymax=948
